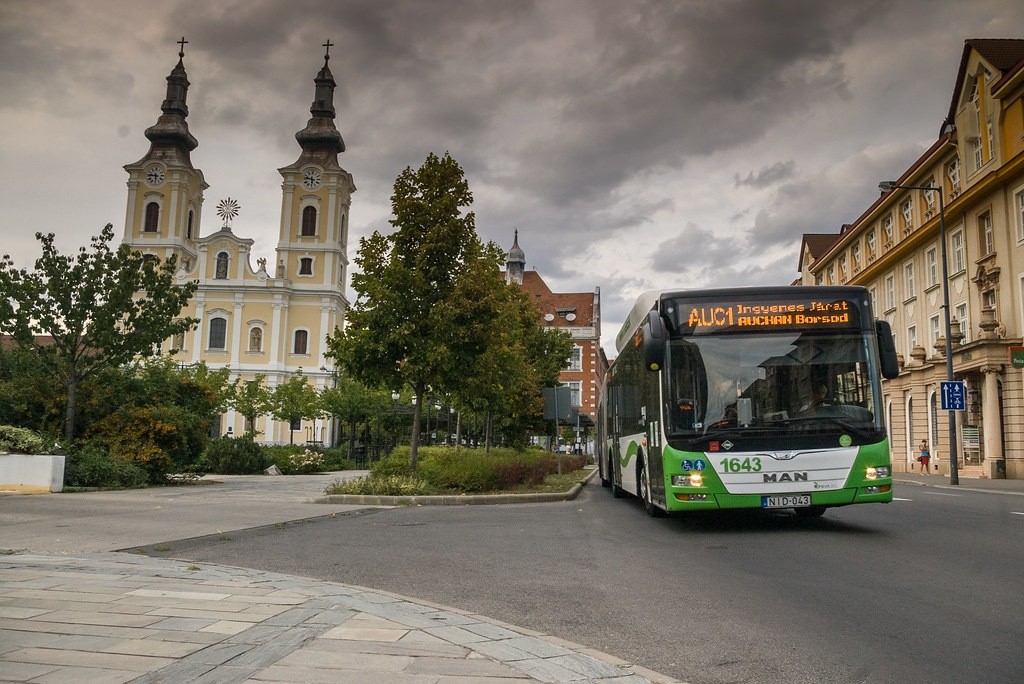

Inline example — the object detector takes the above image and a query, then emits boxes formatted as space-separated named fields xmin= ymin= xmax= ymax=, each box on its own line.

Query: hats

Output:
xmin=922 ymin=439 xmax=927 ymax=442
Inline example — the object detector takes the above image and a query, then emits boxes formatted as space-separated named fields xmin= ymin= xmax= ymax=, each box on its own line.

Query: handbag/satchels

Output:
xmin=918 ymin=457 xmax=921 ymax=461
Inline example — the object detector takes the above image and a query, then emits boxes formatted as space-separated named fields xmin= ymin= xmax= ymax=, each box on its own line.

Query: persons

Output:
xmin=919 ymin=439 xmax=930 ymax=476
xmin=798 ymin=383 xmax=832 ymax=412
xmin=565 ymin=442 xmax=573 ymax=455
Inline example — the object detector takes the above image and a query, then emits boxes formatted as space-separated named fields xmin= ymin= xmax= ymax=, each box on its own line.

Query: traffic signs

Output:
xmin=939 ymin=381 xmax=966 ymax=412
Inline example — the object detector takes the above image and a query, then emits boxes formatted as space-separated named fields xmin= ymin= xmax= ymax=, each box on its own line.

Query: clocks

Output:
xmin=146 ymin=165 xmax=164 ymax=184
xmin=303 ymin=169 xmax=321 ymax=189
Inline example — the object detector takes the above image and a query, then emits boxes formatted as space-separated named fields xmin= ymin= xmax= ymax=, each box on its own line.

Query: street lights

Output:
xmin=878 ymin=180 xmax=967 ymax=484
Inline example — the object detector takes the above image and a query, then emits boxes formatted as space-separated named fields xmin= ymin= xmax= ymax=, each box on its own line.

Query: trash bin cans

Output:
xmin=991 ymin=459 xmax=1006 ymax=479
xmin=354 ymin=447 xmax=367 ymax=469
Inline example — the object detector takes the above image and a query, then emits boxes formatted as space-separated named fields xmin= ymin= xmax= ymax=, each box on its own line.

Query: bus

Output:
xmin=594 ymin=283 xmax=900 ymax=517
